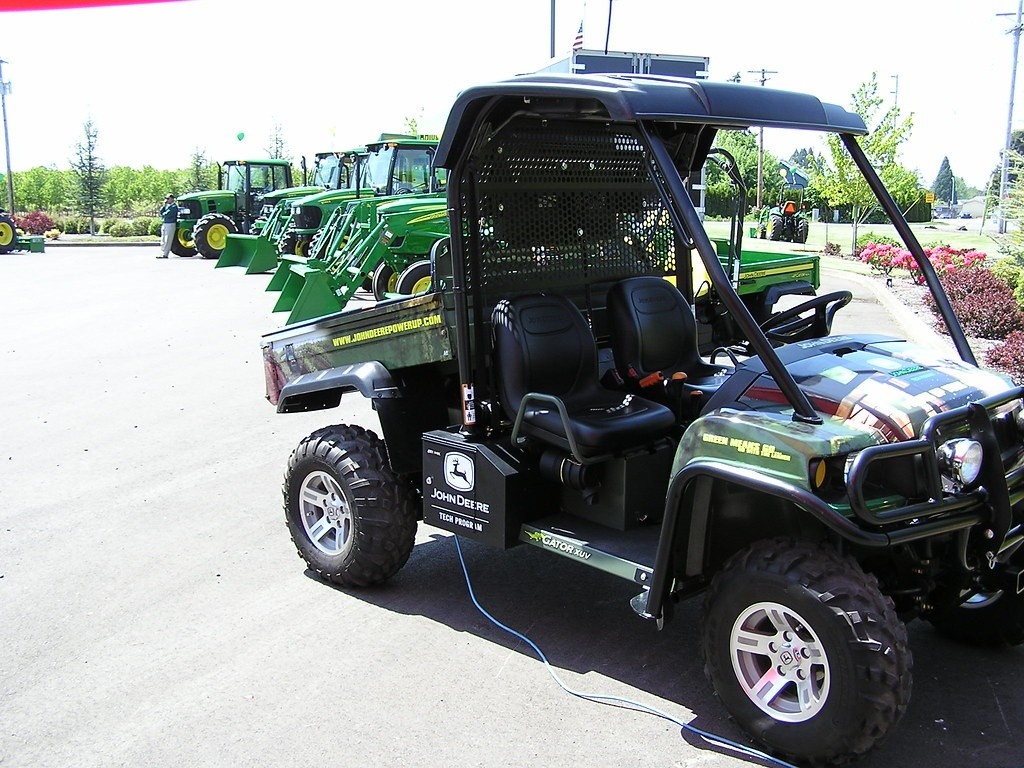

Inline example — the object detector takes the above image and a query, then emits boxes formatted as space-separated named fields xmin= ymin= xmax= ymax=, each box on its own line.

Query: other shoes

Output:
xmin=156 ymin=255 xmax=168 ymax=259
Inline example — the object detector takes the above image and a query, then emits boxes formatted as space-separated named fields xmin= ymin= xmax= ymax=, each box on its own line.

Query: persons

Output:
xmin=156 ymin=193 xmax=178 ymax=259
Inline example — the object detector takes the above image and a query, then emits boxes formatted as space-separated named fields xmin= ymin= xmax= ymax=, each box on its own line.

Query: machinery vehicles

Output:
xmin=260 ymin=52 xmax=1023 ymax=768
xmin=169 ymin=132 xmax=821 ymax=351
xmin=0 ymin=206 xmax=45 ymax=254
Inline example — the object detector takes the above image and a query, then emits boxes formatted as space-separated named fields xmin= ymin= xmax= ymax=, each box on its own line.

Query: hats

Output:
xmin=165 ymin=194 xmax=175 ymax=199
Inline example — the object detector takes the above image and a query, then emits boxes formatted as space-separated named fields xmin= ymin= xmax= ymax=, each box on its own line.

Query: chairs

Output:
xmin=489 ymin=292 xmax=678 ymax=465
xmin=609 ymin=277 xmax=736 ymax=411
